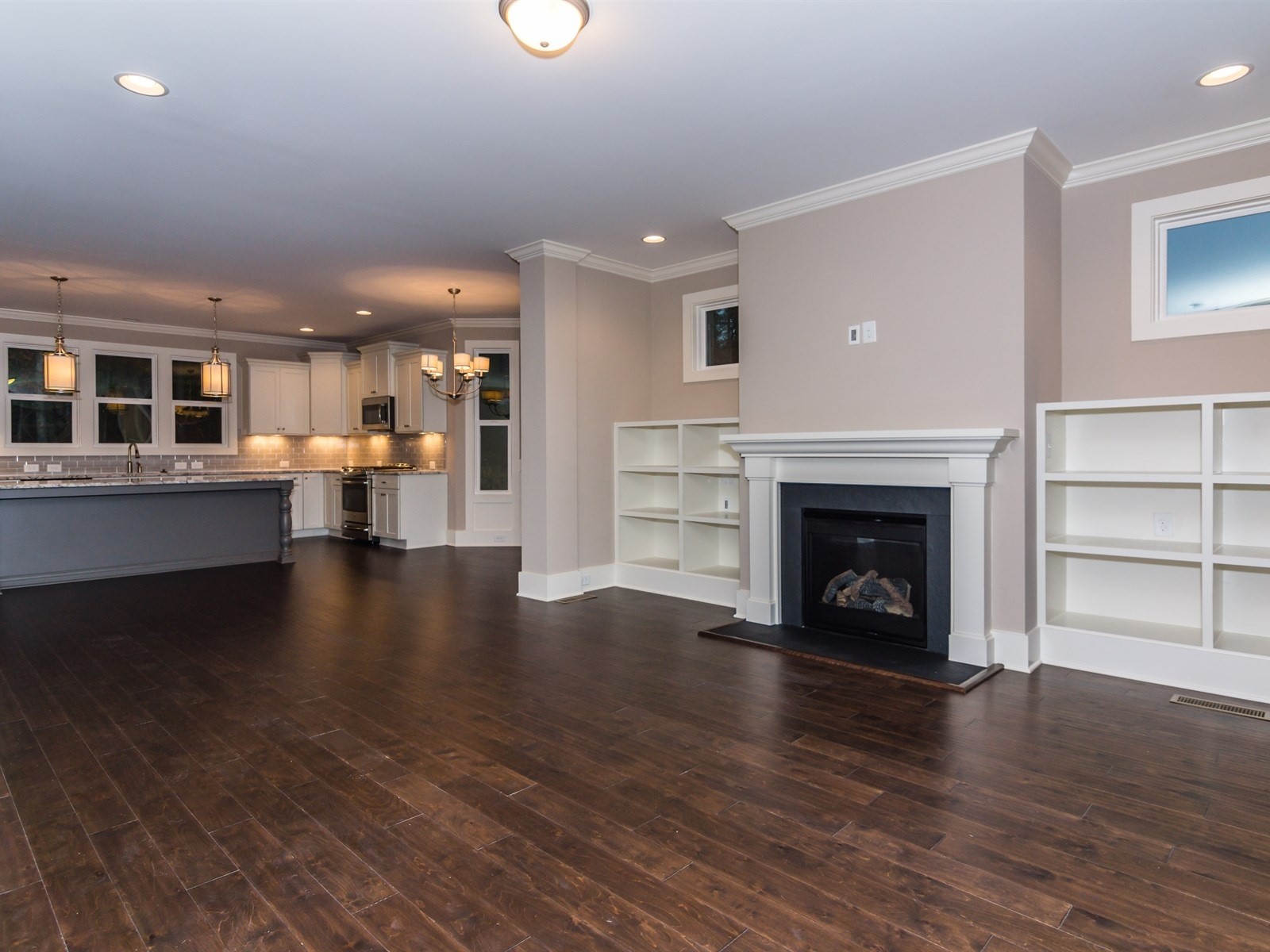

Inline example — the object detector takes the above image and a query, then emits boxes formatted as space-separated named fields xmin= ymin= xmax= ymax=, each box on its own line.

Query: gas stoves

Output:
xmin=342 ymin=463 xmax=416 ymax=476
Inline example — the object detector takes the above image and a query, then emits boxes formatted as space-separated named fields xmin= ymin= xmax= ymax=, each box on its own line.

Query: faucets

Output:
xmin=127 ymin=443 xmax=142 ymax=473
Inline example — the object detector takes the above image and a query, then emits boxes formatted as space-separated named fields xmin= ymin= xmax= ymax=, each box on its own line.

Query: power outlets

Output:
xmin=580 ymin=575 xmax=591 ymax=586
xmin=349 ymin=460 xmax=354 ymax=466
xmin=279 ymin=461 xmax=290 ymax=467
xmin=47 ymin=464 xmax=62 ymax=473
xmin=862 ymin=320 xmax=876 ymax=344
xmin=429 ymin=461 xmax=435 ymax=470
xmin=377 ymin=460 xmax=382 ymax=466
xmin=174 ymin=462 xmax=188 ymax=470
xmin=191 ymin=461 xmax=203 ymax=469
xmin=24 ymin=464 xmax=40 ymax=473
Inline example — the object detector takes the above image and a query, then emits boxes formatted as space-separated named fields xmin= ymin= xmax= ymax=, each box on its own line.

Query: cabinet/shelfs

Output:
xmin=371 ymin=474 xmax=449 ymax=550
xmin=306 ymin=350 xmax=360 ymax=438
xmin=238 ymin=357 xmax=311 ymax=437
xmin=356 ymin=338 xmax=422 ymax=399
xmin=325 ymin=473 xmax=355 ymax=540
xmin=344 ymin=359 xmax=369 ymax=436
xmin=391 ymin=347 xmax=448 ymax=436
xmin=1035 ymin=390 xmax=1270 ymax=707
xmin=281 ymin=472 xmax=328 ymax=538
xmin=612 ymin=417 xmax=742 ymax=609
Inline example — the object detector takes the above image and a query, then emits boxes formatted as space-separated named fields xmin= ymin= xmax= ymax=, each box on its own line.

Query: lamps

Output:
xmin=499 ymin=0 xmax=590 ymax=54
xmin=43 ymin=275 xmax=81 ymax=394
xmin=480 ymin=374 xmax=510 ymax=418
xmin=199 ymin=296 xmax=231 ymax=399
xmin=420 ymin=288 xmax=490 ymax=401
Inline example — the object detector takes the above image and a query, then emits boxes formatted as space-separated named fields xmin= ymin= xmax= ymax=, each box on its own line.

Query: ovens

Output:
xmin=361 ymin=396 xmax=395 ymax=434
xmin=340 ymin=472 xmax=374 ymax=542
